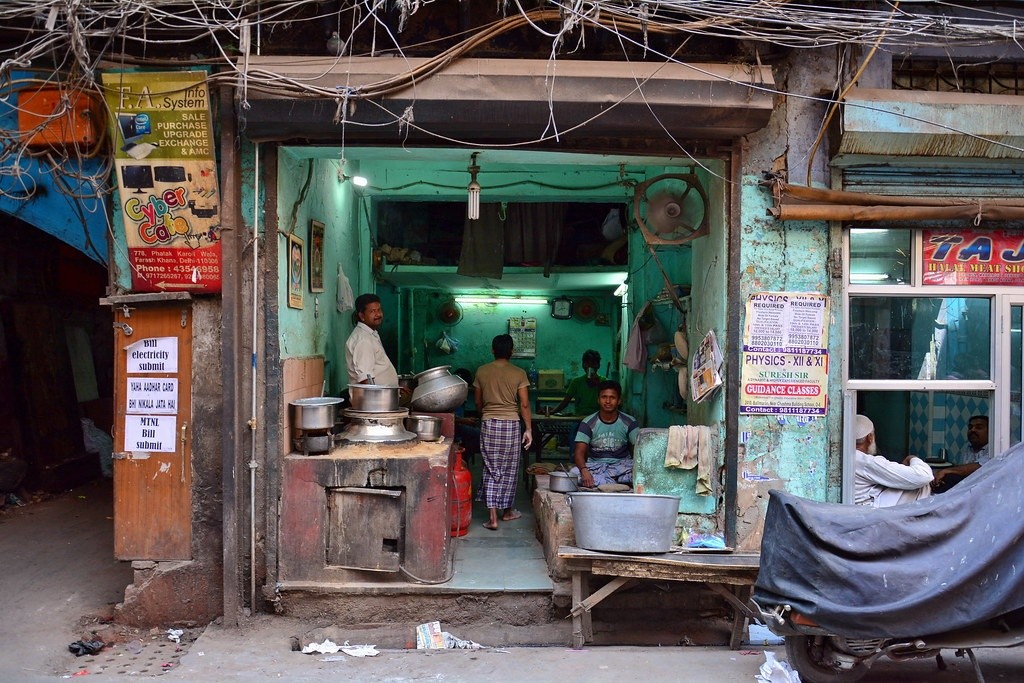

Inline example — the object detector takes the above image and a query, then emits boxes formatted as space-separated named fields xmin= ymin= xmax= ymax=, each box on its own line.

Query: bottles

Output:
xmin=529 ymin=361 xmax=536 ymax=389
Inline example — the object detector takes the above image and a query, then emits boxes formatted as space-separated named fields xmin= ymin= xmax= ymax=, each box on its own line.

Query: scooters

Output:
xmin=747 ymin=438 xmax=1024 ymax=683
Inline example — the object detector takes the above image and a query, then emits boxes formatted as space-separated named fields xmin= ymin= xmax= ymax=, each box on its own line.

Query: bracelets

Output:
xmin=580 ymin=466 xmax=587 ymax=472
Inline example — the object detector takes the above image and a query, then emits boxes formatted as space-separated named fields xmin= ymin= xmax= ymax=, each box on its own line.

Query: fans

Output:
xmin=572 ymin=295 xmax=601 ymax=323
xmin=435 ymin=299 xmax=464 ymax=328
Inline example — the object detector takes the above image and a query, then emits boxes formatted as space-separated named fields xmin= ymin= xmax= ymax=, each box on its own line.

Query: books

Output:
xmin=690 ymin=330 xmax=725 ymax=403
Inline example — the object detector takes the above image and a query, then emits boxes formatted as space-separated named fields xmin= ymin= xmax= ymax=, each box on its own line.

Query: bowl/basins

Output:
xmin=564 ymin=490 xmax=682 ymax=553
xmin=548 ymin=471 xmax=579 ymax=492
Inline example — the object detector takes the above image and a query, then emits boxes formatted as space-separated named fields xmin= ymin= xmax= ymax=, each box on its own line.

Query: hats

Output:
xmin=857 ymin=414 xmax=874 ymax=440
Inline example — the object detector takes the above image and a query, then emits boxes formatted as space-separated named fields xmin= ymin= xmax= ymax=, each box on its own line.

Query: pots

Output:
xmin=405 ymin=415 xmax=446 ymax=442
xmin=347 ymin=384 xmax=403 ymax=412
xmin=411 ymin=365 xmax=469 ymax=413
xmin=288 ymin=397 xmax=345 ymax=430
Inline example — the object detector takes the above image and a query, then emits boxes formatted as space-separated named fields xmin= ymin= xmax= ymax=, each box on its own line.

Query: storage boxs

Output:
xmin=538 ymin=370 xmax=564 ymax=390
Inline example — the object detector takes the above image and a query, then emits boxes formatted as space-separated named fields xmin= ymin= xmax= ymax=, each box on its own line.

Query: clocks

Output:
xmin=551 ymin=295 xmax=573 ymax=320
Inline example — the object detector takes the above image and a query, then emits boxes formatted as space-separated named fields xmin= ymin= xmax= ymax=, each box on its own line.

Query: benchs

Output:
xmin=557 ymin=544 xmax=758 ymax=649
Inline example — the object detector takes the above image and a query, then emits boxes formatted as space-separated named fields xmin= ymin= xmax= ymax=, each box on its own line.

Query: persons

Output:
xmin=451 ymin=403 xmax=481 ymax=461
xmin=345 ymin=293 xmax=412 ymax=406
xmin=472 ymin=334 xmax=533 ymax=528
xmin=856 ymin=414 xmax=935 ymax=508
xmin=931 ymin=415 xmax=989 ymax=493
xmin=567 ymin=380 xmax=642 ymax=488
xmin=550 ymin=349 xmax=610 ymax=463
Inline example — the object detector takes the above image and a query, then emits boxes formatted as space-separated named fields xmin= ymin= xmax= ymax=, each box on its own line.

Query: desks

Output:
xmin=528 ymin=397 xmax=587 ymax=465
